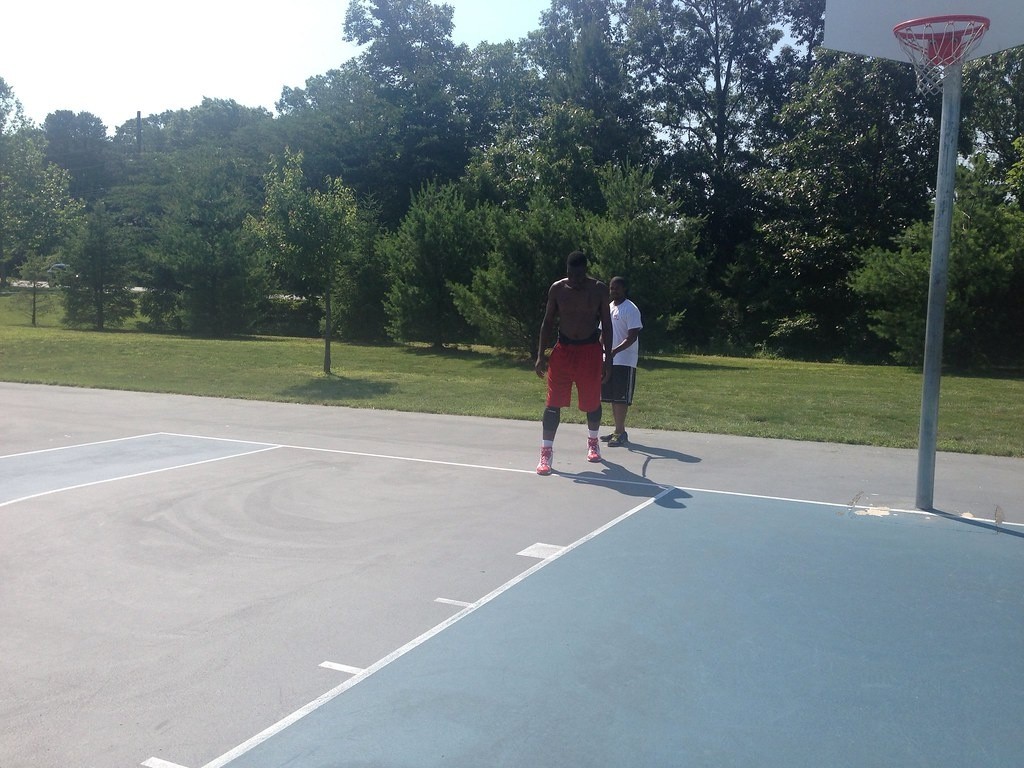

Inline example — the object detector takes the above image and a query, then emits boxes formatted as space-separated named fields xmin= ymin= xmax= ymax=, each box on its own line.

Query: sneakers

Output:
xmin=600 ymin=432 xmax=628 ymax=447
xmin=587 ymin=437 xmax=601 ymax=464
xmin=535 ymin=447 xmax=554 ymax=476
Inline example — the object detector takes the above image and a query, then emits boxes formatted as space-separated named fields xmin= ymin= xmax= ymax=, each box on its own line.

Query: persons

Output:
xmin=531 ymin=252 xmax=614 ymax=475
xmin=597 ymin=277 xmax=644 ymax=447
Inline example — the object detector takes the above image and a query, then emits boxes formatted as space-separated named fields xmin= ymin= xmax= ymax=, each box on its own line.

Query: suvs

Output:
xmin=46 ymin=265 xmax=90 ymax=289
